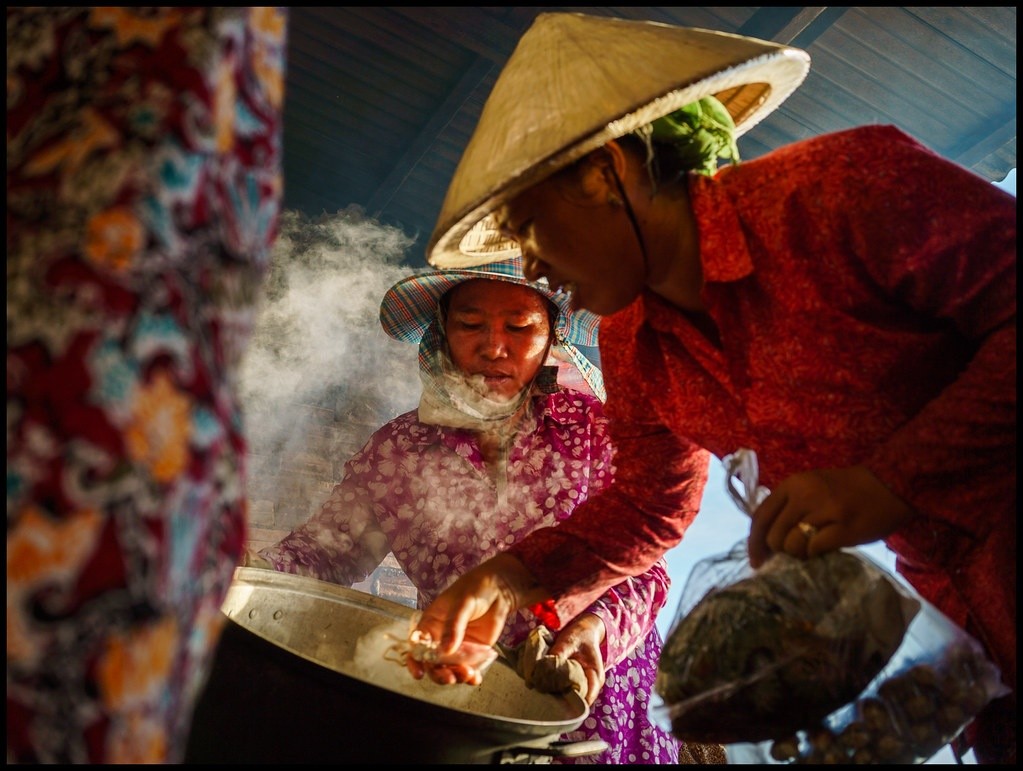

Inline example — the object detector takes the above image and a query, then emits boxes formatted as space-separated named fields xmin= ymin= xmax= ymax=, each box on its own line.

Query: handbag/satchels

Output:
xmin=646 ymin=450 xmax=1002 ymax=765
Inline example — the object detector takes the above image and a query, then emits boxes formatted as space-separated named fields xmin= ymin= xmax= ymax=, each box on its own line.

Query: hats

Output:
xmin=379 ymin=252 xmax=609 ymax=409
xmin=424 ymin=10 xmax=810 ymax=270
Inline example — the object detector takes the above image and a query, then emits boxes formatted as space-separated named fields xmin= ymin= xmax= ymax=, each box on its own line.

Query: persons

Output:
xmin=240 ymin=254 xmax=685 ymax=763
xmin=407 ymin=12 xmax=1023 ymax=764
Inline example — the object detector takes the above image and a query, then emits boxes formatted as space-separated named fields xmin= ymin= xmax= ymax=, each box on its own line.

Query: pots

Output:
xmin=188 ymin=566 xmax=591 ymax=764
